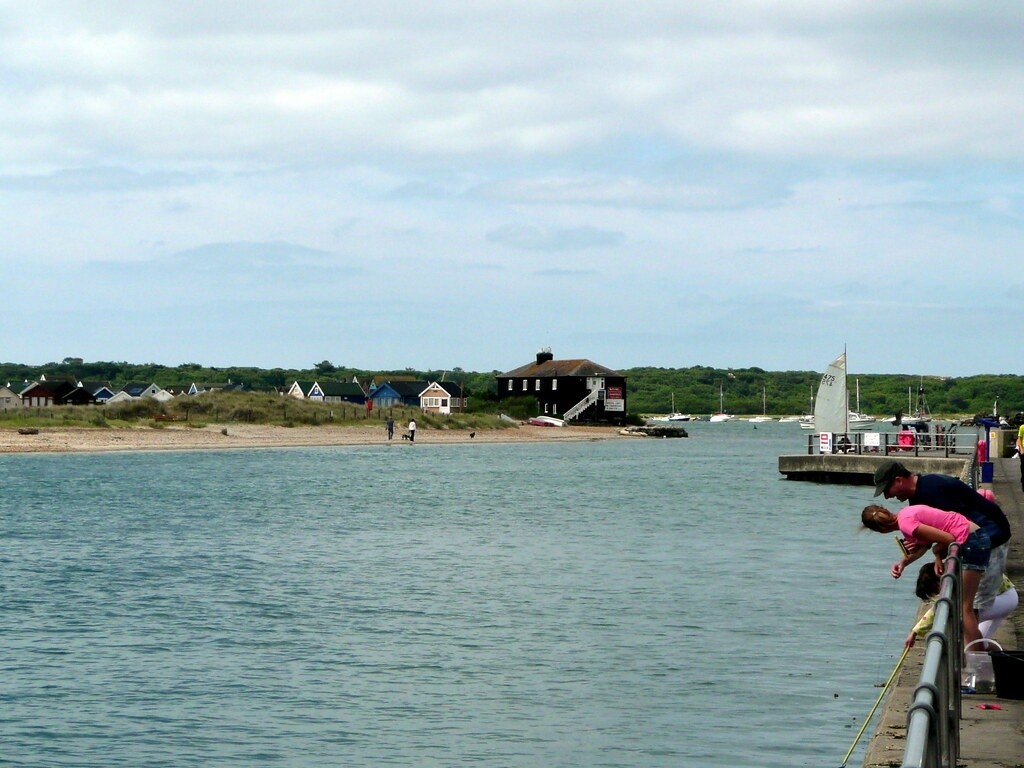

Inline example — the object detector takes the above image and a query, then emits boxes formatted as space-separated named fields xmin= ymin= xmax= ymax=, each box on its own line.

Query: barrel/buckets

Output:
xmin=967 ymin=651 xmax=996 ymax=691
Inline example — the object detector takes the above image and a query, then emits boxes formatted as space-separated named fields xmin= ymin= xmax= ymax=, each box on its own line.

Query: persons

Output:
xmin=408 ymin=419 xmax=417 ymax=442
xmin=837 ymin=438 xmax=852 ymax=452
xmin=1018 ymin=424 xmax=1024 ymax=494
xmin=385 ymin=416 xmax=395 ymax=440
xmin=898 ymin=425 xmax=921 ymax=451
xmin=862 ymin=460 xmax=1019 ymax=667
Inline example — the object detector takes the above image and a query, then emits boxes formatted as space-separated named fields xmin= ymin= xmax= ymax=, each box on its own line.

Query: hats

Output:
xmin=873 ymin=460 xmax=902 ymax=498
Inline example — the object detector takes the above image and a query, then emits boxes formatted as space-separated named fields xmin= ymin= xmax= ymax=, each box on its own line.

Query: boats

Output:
xmin=747 ymin=407 xmax=931 ymax=429
xmin=669 ymin=412 xmax=690 ymax=421
xmin=709 ymin=413 xmax=730 ymax=422
